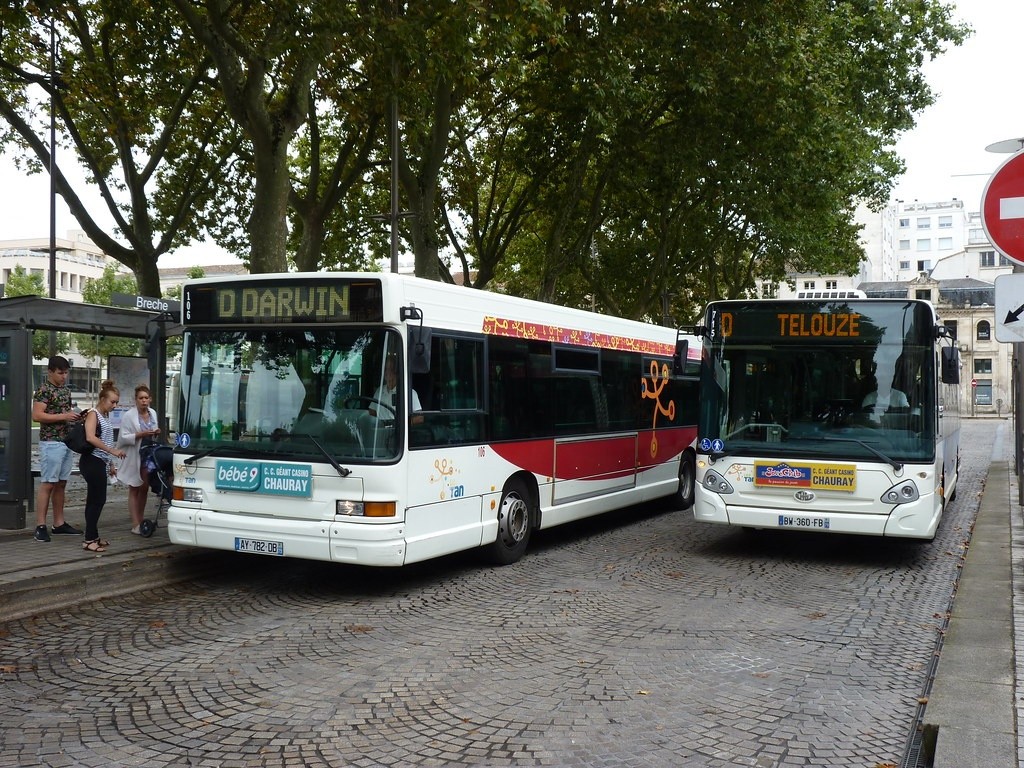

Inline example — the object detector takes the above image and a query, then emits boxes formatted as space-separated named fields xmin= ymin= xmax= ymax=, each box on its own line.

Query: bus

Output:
xmin=673 ymin=298 xmax=962 ymax=542
xmin=168 ymin=271 xmax=703 ymax=567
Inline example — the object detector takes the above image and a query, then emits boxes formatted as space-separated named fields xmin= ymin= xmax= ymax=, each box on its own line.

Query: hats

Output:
xmin=71 ymin=400 xmax=77 ymax=404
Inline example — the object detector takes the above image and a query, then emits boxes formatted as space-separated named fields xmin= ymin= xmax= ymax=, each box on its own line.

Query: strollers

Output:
xmin=139 ymin=430 xmax=173 ymax=538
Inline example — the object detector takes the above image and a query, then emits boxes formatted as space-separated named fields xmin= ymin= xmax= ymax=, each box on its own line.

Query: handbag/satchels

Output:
xmin=63 ymin=411 xmax=102 ymax=456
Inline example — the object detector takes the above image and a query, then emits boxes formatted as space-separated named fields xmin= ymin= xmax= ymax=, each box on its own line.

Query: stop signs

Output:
xmin=980 ymin=148 xmax=1024 ymax=266
xmin=971 ymin=379 xmax=977 ymax=388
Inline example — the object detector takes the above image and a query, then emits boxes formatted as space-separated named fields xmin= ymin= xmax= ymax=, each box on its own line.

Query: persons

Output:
xmin=32 ymin=356 xmax=126 ymax=552
xmin=369 ymin=358 xmax=424 ymax=429
xmin=116 ymin=383 xmax=161 ymax=534
xmin=862 ymin=367 xmax=910 ymax=407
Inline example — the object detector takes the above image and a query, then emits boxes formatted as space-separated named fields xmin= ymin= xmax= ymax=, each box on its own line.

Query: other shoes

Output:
xmin=130 ymin=525 xmax=140 ymax=534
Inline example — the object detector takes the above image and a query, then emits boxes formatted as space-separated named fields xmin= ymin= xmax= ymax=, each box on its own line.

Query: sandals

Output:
xmin=82 ymin=538 xmax=110 ymax=552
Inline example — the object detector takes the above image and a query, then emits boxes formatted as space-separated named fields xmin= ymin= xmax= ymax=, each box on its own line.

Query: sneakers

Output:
xmin=51 ymin=520 xmax=84 ymax=535
xmin=33 ymin=525 xmax=51 ymax=542
